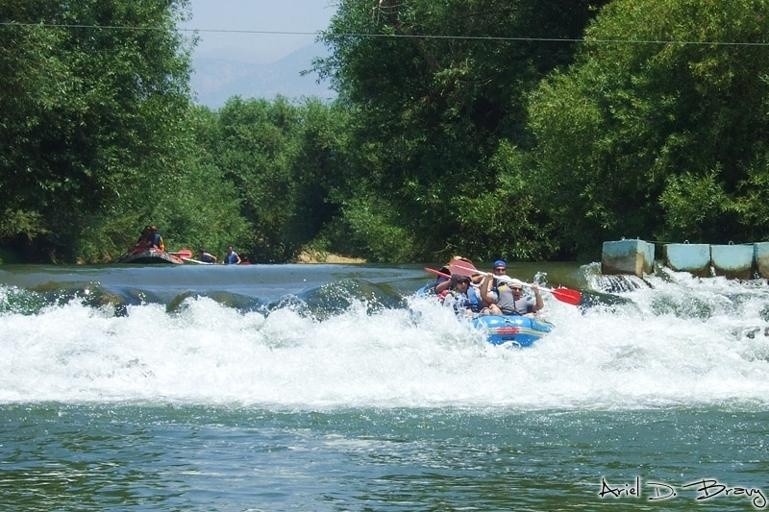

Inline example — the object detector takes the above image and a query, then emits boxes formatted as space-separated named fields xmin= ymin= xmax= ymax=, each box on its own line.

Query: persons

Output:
xmin=148 ymin=226 xmax=165 ymax=250
xmin=139 ymin=226 xmax=150 ymax=242
xmin=198 ymin=249 xmax=217 ymax=263
xmin=435 ymin=256 xmax=543 ymax=320
xmin=224 ymin=245 xmax=241 ymax=264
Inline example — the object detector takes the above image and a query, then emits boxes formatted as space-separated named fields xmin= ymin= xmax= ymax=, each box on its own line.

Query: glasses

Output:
xmin=511 ymin=288 xmax=522 ymax=291
xmin=496 ymin=269 xmax=506 ymax=271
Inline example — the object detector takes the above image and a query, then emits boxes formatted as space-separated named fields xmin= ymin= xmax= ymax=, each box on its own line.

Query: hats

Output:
xmin=454 ymin=256 xmax=472 ymax=263
xmin=496 ymin=275 xmax=522 ymax=287
xmin=494 ymin=260 xmax=505 ymax=269
xmin=456 ymin=274 xmax=469 ymax=282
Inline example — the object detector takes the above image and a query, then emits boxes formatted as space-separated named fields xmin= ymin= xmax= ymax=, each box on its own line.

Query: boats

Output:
xmin=417 ymin=277 xmax=556 ymax=350
xmin=179 ymin=256 xmax=214 ymax=265
xmin=113 ymin=248 xmax=184 ymax=265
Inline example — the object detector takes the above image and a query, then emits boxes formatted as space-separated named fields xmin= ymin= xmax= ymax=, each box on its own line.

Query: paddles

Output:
xmin=425 ymin=267 xmax=449 ymax=278
xmin=168 ymin=249 xmax=192 ymax=258
xmin=449 ymin=260 xmax=580 ymax=305
